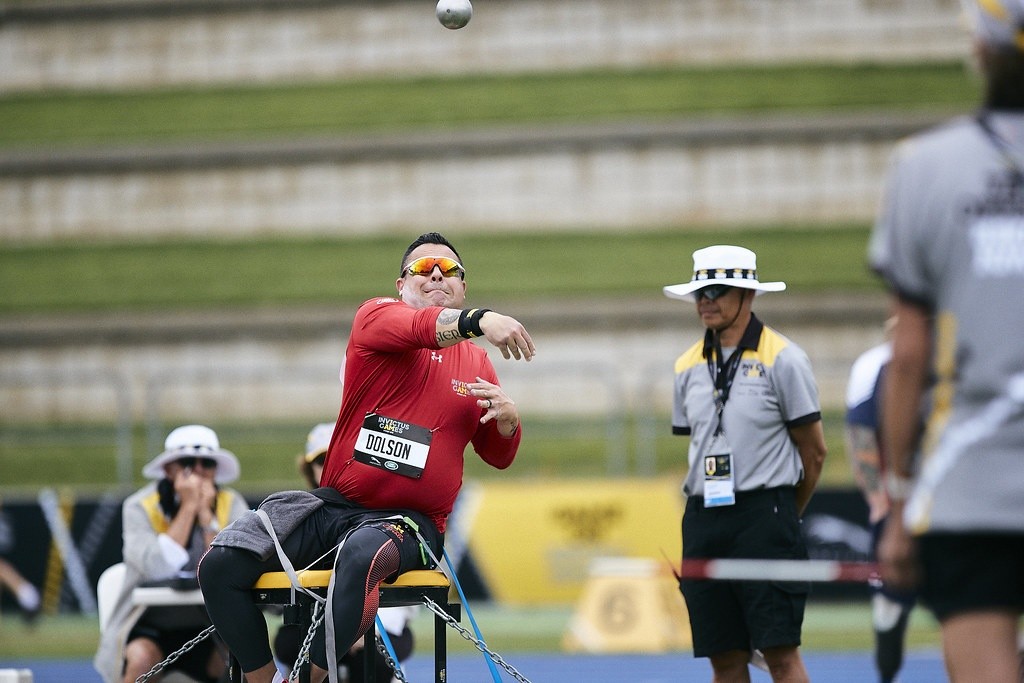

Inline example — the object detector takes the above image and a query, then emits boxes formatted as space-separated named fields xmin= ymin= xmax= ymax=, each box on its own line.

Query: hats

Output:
xmin=305 ymin=422 xmax=337 ymax=463
xmin=142 ymin=425 xmax=239 ymax=485
xmin=663 ymin=245 xmax=787 ymax=303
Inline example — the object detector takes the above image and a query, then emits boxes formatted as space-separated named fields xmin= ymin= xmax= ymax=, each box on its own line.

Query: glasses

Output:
xmin=401 ymin=256 xmax=465 ymax=281
xmin=177 ymin=457 xmax=216 ymax=468
xmin=314 ymin=455 xmax=325 ymax=465
xmin=695 ymin=284 xmax=732 ymax=299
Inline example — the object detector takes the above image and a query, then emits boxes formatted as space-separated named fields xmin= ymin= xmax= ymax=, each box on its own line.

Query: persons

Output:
xmin=91 ymin=425 xmax=250 ymax=683
xmin=847 ymin=313 xmax=937 ymax=683
xmin=862 ymin=0 xmax=1024 ymax=683
xmin=198 ymin=232 xmax=536 ymax=683
xmin=273 ymin=422 xmax=413 ymax=683
xmin=664 ymin=246 xmax=828 ymax=683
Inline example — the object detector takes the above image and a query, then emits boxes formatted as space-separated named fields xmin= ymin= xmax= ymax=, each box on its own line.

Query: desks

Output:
xmin=135 ymin=587 xmax=208 ymax=607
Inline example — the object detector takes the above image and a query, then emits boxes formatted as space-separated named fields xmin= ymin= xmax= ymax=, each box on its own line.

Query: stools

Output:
xmin=227 ymin=569 xmax=460 ymax=682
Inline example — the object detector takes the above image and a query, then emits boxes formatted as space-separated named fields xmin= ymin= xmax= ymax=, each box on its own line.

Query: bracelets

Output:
xmin=200 ymin=517 xmax=219 ymax=533
xmin=487 ymin=399 xmax=492 ymax=408
xmin=458 ymin=308 xmax=493 ymax=339
xmin=882 ymin=476 xmax=914 ymax=501
